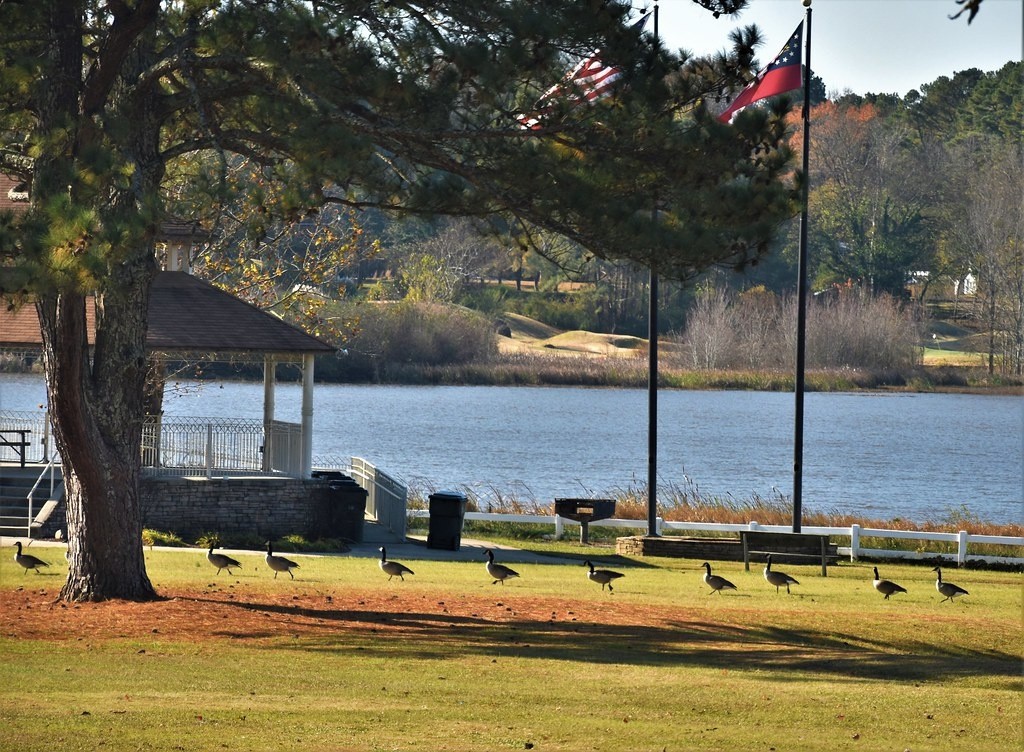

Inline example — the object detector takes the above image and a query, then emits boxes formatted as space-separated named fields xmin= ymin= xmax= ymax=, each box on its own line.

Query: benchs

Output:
xmin=0 ymin=441 xmax=31 ymax=468
xmin=739 ymin=530 xmax=839 ymax=577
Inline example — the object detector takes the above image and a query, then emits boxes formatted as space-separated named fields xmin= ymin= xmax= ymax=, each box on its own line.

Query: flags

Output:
xmin=715 ymin=18 xmax=806 ymax=129
xmin=513 ymin=7 xmax=654 ymax=141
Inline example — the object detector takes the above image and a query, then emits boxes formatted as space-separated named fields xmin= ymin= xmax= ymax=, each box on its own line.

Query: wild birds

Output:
xmin=700 ymin=562 xmax=737 ymax=595
xmin=263 ymin=541 xmax=301 ymax=580
xmin=12 ymin=541 xmax=50 ymax=576
xmin=377 ymin=546 xmax=415 ymax=581
xmin=482 ymin=549 xmax=520 ymax=585
xmin=203 ymin=539 xmax=243 ymax=576
xmin=932 ymin=567 xmax=970 ymax=603
xmin=582 ymin=560 xmax=625 ymax=591
xmin=872 ymin=567 xmax=907 ymax=600
xmin=761 ymin=553 xmax=798 ymax=592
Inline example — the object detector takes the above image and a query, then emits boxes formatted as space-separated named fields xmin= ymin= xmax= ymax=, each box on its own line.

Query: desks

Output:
xmin=0 ymin=429 xmax=31 ymax=468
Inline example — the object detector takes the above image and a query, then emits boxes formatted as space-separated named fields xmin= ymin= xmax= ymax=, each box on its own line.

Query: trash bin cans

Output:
xmin=427 ymin=490 xmax=469 ymax=552
